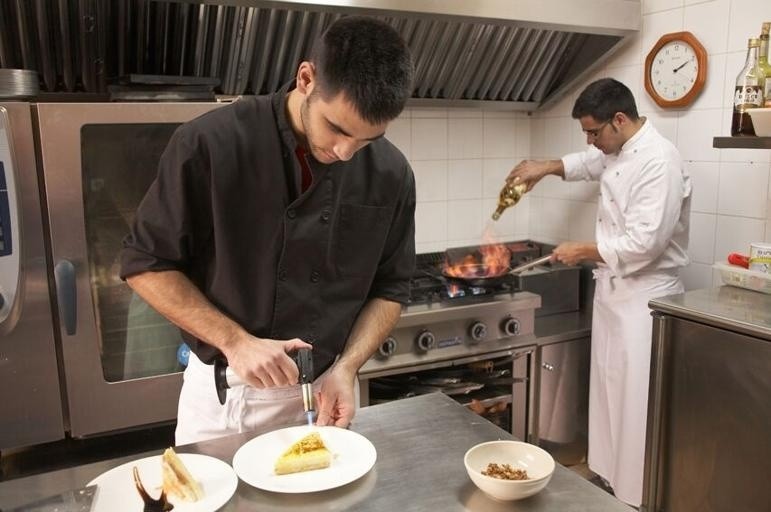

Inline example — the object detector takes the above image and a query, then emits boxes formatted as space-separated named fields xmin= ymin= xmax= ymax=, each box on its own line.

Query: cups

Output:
xmin=3 ymin=69 xmax=44 ymax=100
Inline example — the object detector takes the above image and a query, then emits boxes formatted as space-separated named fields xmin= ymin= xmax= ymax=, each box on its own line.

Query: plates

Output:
xmin=233 ymin=424 xmax=379 ymax=494
xmin=86 ymin=452 xmax=239 ymax=512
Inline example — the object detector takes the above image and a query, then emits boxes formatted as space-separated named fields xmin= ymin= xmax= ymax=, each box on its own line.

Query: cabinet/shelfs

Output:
xmin=636 ymin=283 xmax=771 ymax=511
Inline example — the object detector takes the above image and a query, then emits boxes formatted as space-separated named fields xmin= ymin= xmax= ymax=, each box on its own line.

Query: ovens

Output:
xmin=370 ymin=350 xmax=533 ymax=448
xmin=1 ymin=89 xmax=245 ymax=455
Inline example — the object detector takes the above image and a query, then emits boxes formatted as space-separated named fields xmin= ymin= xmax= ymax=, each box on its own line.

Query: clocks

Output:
xmin=642 ymin=30 xmax=711 ymax=112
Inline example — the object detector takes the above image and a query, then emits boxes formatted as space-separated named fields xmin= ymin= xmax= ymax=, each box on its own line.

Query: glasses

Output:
xmin=581 ymin=118 xmax=612 ymax=139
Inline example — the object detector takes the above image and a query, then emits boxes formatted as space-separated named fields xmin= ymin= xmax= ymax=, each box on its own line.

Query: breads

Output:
xmin=162 ymin=448 xmax=202 ymax=503
xmin=275 ymin=432 xmax=330 ymax=476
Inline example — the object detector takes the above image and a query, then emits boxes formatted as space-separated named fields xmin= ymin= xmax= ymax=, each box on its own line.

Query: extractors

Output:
xmin=0 ymin=0 xmax=644 ymax=118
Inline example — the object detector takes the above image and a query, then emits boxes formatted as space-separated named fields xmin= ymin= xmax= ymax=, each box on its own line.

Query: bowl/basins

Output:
xmin=464 ymin=439 xmax=558 ymax=501
xmin=745 ymin=109 xmax=771 ymax=137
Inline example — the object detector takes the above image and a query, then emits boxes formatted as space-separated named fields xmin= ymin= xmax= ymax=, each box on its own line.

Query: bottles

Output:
xmin=731 ymin=39 xmax=766 ymax=137
xmin=760 ymin=21 xmax=771 ymax=108
xmin=491 ymin=172 xmax=542 ymax=223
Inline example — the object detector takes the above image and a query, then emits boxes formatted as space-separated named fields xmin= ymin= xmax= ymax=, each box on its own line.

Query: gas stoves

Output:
xmin=372 ymin=260 xmax=542 ymax=360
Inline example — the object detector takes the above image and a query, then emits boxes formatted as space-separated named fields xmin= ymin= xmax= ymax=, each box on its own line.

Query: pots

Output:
xmin=441 ymin=254 xmax=552 ymax=286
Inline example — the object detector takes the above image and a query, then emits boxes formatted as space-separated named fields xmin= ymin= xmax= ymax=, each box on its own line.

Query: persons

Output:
xmin=506 ymin=78 xmax=690 ymax=508
xmin=119 ymin=18 xmax=417 ymax=447
